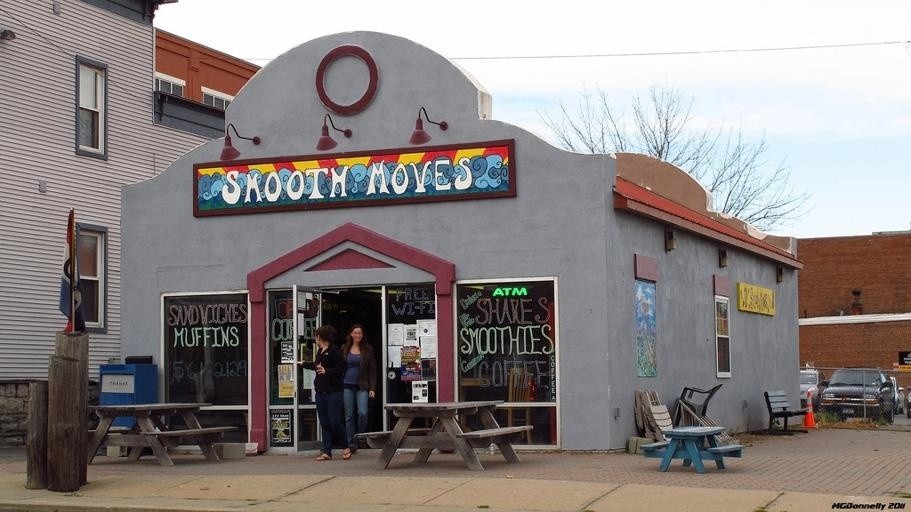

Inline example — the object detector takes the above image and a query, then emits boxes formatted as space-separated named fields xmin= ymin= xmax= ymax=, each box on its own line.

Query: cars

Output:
xmin=819 ymin=365 xmax=905 ymax=424
xmin=800 ymin=368 xmax=826 ymax=413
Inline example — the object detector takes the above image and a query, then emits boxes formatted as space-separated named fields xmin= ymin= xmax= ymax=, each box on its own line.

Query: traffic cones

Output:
xmin=800 ymin=390 xmax=818 ymax=428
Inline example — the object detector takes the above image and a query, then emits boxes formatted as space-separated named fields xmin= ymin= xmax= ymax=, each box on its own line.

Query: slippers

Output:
xmin=316 ymin=455 xmax=332 ymax=460
xmin=342 ymin=448 xmax=351 ymax=460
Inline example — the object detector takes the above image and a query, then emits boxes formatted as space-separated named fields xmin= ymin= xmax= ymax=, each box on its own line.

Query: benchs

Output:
xmin=641 ymin=440 xmax=746 ymax=473
xmin=354 ymin=424 xmax=535 ymax=470
xmin=86 ymin=426 xmax=238 ymax=466
xmin=763 ymin=390 xmax=810 ymax=436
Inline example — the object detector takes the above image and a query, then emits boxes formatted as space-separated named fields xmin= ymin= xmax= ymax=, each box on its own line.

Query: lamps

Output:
xmin=222 ymin=122 xmax=261 ymax=159
xmin=408 ymin=107 xmax=449 ymax=145
xmin=316 ymin=113 xmax=352 ymax=152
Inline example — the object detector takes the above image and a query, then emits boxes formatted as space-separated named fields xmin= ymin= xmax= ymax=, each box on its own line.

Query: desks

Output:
xmin=86 ymin=403 xmax=221 ymax=466
xmin=374 ymin=401 xmax=521 ymax=469
xmin=659 ymin=426 xmax=727 ymax=473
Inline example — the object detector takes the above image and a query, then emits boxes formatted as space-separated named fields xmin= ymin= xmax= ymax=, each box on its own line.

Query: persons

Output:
xmin=341 ymin=325 xmax=377 ymax=453
xmin=297 ymin=325 xmax=353 ymax=460
